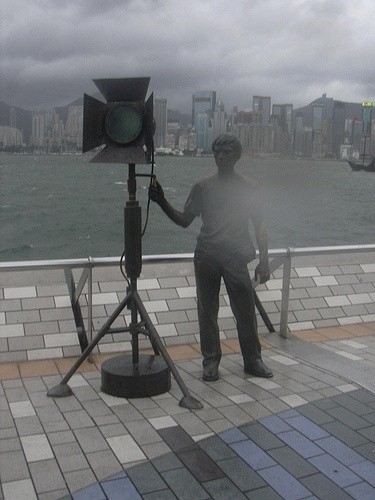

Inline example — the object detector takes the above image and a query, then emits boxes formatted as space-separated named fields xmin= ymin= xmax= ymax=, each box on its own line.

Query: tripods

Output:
xmin=46 ymin=164 xmax=203 ymax=409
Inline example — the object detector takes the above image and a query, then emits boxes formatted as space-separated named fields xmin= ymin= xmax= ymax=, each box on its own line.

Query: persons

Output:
xmin=149 ymin=132 xmax=273 ymax=382
